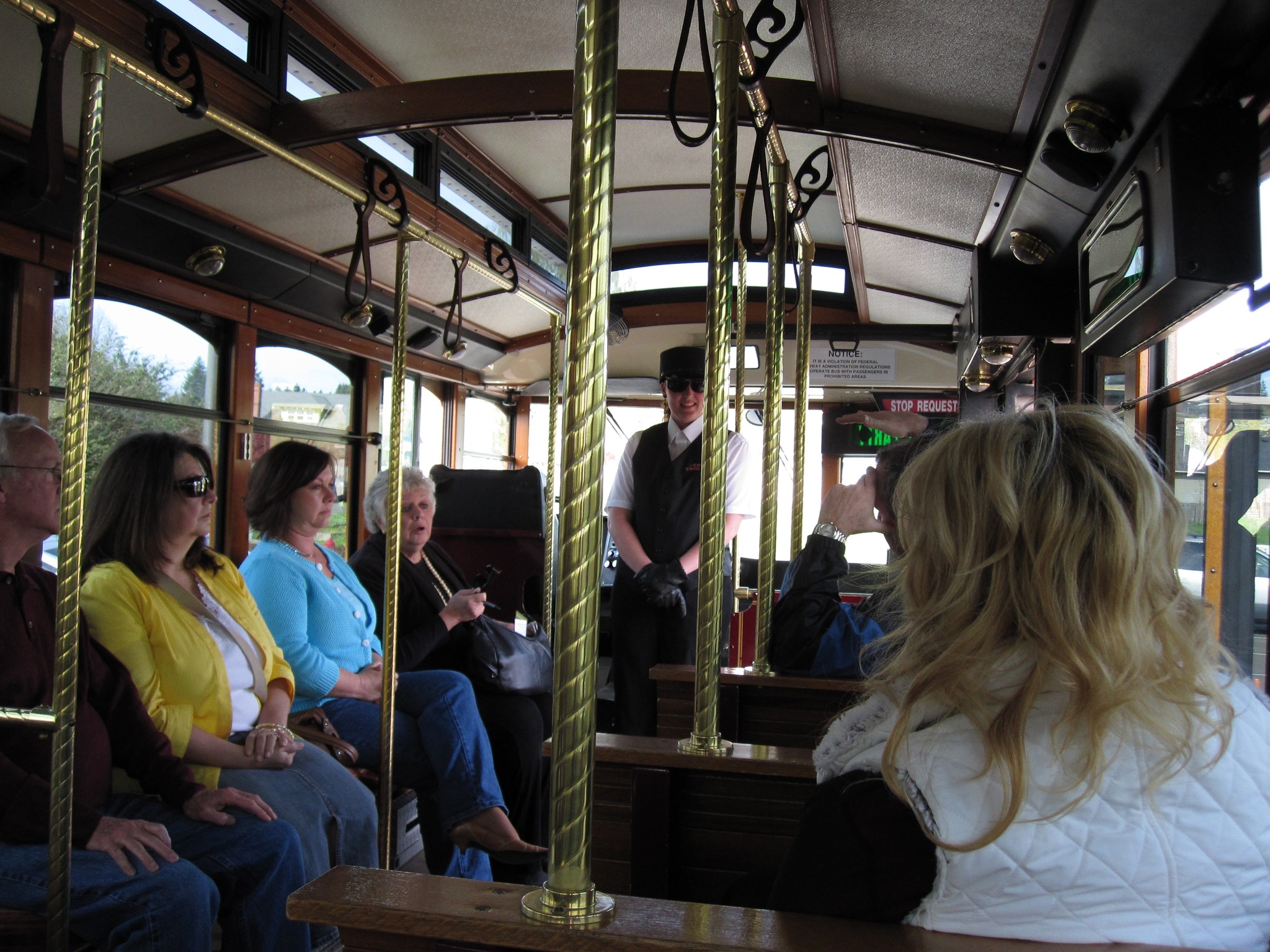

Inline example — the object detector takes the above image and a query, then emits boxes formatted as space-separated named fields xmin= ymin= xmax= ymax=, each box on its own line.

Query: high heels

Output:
xmin=449 ymin=819 xmax=552 ymax=859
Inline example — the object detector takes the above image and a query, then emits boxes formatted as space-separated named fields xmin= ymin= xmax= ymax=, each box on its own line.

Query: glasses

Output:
xmin=662 ymin=374 xmax=708 ymax=394
xmin=167 ymin=474 xmax=214 ymax=497
xmin=0 ymin=460 xmax=62 ymax=480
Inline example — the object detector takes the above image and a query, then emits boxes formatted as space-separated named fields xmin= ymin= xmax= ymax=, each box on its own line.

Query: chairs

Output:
xmin=428 ymin=463 xmax=545 ymax=531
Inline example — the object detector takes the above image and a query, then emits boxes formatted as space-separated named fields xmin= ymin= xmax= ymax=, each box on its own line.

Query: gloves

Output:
xmin=633 ymin=563 xmax=688 ymax=616
xmin=634 ymin=554 xmax=687 ymax=603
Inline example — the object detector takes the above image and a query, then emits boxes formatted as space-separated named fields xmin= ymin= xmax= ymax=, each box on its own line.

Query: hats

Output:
xmin=660 ymin=345 xmax=709 ymax=378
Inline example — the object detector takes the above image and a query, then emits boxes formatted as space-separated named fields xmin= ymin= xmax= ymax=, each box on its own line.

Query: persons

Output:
xmin=347 ymin=466 xmax=553 ymax=870
xmin=1 ymin=410 xmax=315 ymax=952
xmin=765 ymin=410 xmax=964 ymax=686
xmin=763 ymin=402 xmax=1269 ymax=951
xmin=74 ymin=431 xmax=381 ymax=952
xmin=237 ymin=439 xmax=550 ymax=882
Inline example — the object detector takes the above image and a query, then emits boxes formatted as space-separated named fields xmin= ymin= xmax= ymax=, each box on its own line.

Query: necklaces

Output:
xmin=418 ymin=546 xmax=453 ymax=607
xmin=273 ymin=535 xmax=314 ymax=559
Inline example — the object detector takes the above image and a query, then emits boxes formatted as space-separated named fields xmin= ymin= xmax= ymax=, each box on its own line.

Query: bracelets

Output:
xmin=253 ymin=722 xmax=294 ymax=741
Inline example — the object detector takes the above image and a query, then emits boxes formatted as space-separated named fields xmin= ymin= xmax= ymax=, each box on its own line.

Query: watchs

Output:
xmin=812 ymin=521 xmax=847 ymax=545
xmin=603 ymin=344 xmax=760 ymax=739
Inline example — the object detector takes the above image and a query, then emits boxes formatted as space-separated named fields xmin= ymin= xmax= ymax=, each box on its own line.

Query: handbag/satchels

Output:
xmin=286 ymin=705 xmax=357 ymax=767
xmin=465 ymin=613 xmax=555 ymax=695
